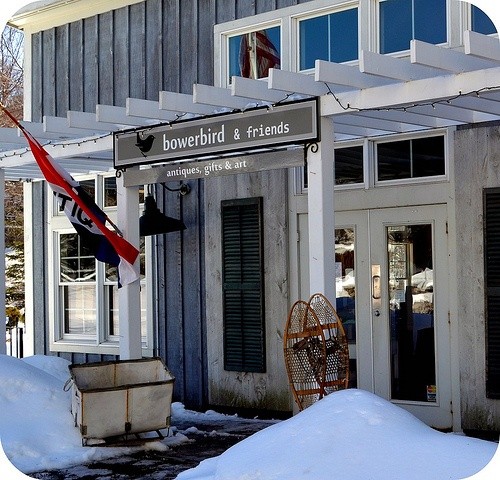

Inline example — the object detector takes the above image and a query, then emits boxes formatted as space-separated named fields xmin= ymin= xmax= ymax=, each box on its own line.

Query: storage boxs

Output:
xmin=63 ymin=357 xmax=175 ymax=446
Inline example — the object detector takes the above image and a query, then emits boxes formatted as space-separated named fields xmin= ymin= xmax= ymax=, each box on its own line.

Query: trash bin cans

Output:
xmin=63 ymin=356 xmax=175 ymax=447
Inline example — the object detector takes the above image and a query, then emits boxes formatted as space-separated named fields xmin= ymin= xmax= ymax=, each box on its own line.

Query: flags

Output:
xmin=1 ymin=104 xmax=141 ymax=290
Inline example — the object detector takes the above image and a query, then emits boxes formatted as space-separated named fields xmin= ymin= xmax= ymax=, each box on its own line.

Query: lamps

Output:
xmin=140 ymin=182 xmax=190 ymax=236
